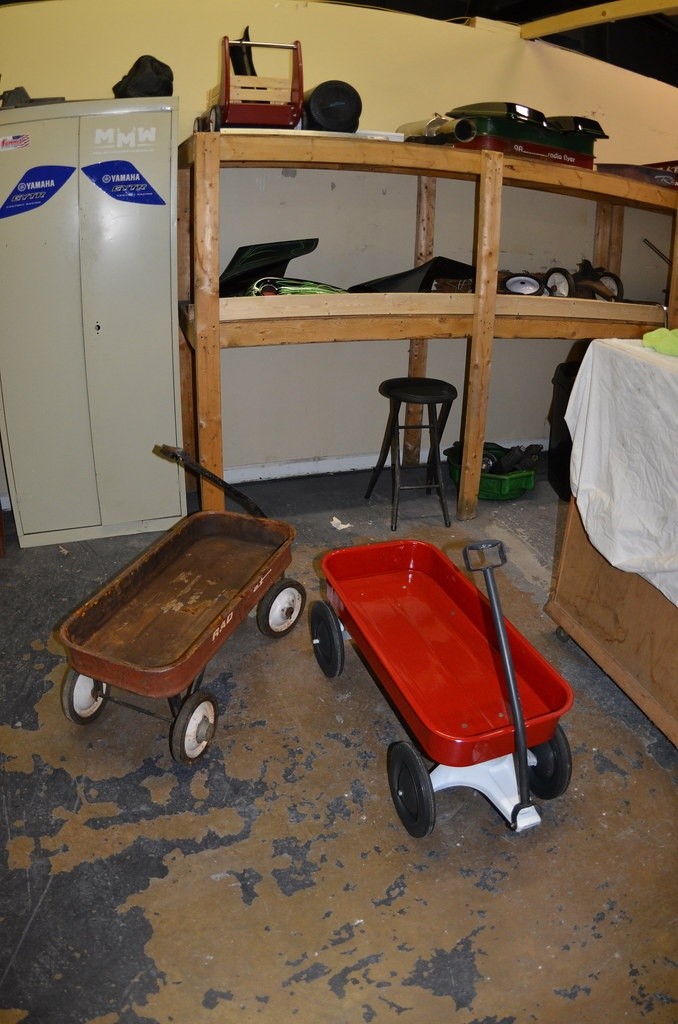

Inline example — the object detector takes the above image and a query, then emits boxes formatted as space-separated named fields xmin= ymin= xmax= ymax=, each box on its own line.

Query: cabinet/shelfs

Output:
xmin=177 ymin=123 xmax=678 ymax=525
xmin=0 ymin=97 xmax=189 ymax=552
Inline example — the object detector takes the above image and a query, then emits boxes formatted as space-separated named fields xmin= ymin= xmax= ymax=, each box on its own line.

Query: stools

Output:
xmin=366 ymin=375 xmax=459 ymax=530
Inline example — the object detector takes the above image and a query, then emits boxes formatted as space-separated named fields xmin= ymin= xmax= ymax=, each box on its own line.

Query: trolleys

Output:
xmin=54 ymin=444 xmax=306 ymax=767
xmin=308 ymin=539 xmax=572 ymax=839
